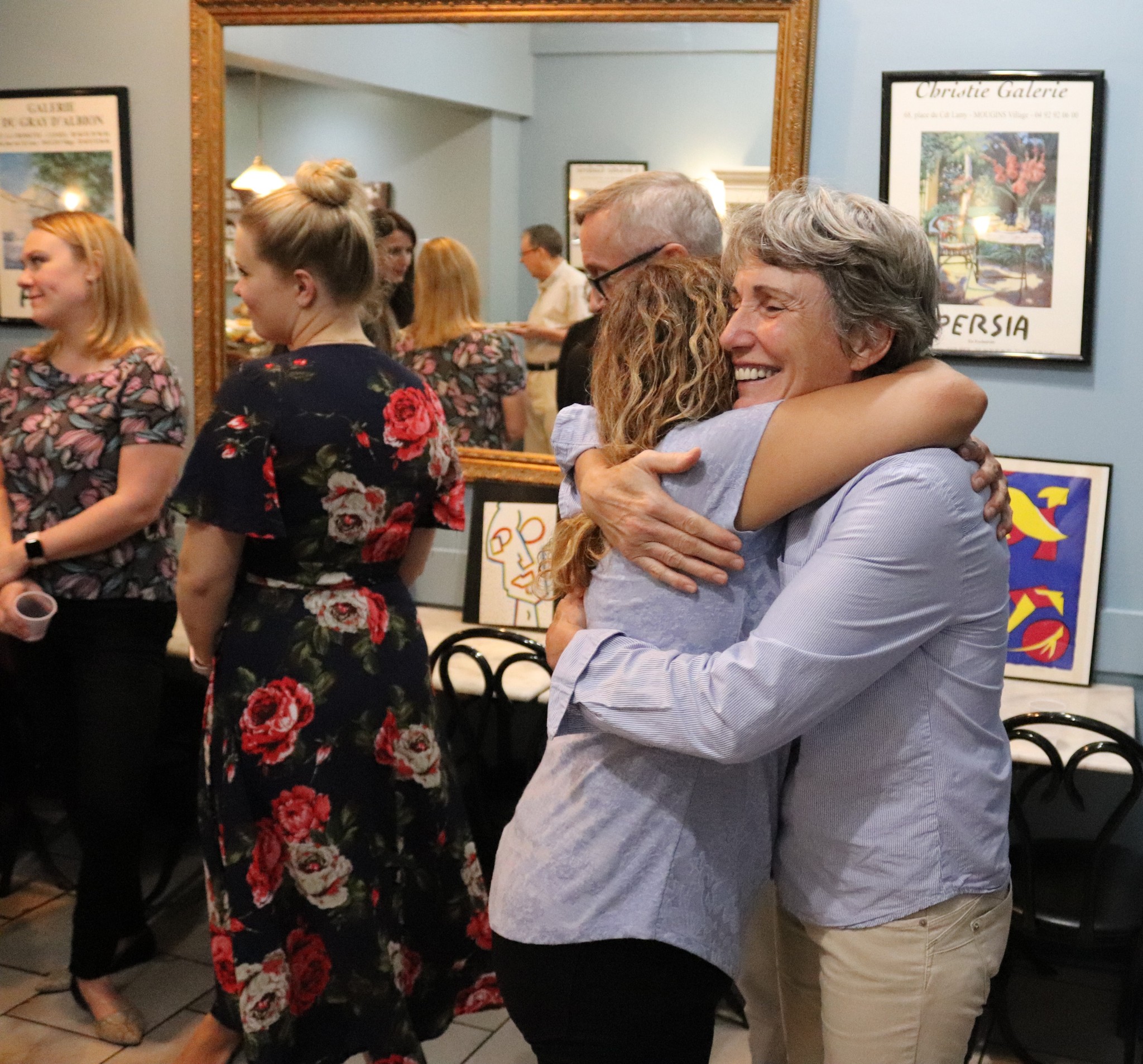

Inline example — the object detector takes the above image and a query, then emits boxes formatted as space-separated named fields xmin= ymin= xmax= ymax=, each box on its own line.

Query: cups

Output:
xmin=14 ymin=591 xmax=58 ymax=641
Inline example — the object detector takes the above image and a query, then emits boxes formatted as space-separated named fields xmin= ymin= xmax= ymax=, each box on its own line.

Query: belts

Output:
xmin=525 ymin=361 xmax=558 ymax=371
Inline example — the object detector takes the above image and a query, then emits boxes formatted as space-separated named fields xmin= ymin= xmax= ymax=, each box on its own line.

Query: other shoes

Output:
xmin=69 ymin=975 xmax=144 ymax=1046
xmin=36 ymin=965 xmax=140 ymax=995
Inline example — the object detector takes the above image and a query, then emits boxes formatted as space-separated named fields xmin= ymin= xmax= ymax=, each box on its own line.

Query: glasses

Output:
xmin=586 ymin=243 xmax=666 ymax=303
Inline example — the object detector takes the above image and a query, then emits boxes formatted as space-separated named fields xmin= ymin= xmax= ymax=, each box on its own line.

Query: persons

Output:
xmin=503 ymin=225 xmax=596 ymax=460
xmin=450 ymin=157 xmax=1018 ymax=1063
xmin=389 ymin=233 xmax=527 ymax=453
xmin=361 ymin=209 xmax=417 ymax=365
xmin=168 ymin=158 xmax=506 ymax=1064
xmin=0 ymin=212 xmax=194 ymax=1047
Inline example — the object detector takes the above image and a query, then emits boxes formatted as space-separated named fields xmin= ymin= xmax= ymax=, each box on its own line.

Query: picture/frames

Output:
xmin=992 ymin=455 xmax=1114 ymax=687
xmin=567 ymin=160 xmax=648 ymax=272
xmin=880 ymin=70 xmax=1105 ymax=367
xmin=0 ymin=86 xmax=135 ymax=329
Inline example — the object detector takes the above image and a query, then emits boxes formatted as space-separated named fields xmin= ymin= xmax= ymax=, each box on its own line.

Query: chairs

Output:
xmin=431 ymin=628 xmax=555 ymax=894
xmin=973 ymin=712 xmax=1143 ymax=1064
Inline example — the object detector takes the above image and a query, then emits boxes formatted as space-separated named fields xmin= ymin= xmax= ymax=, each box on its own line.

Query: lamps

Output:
xmin=231 ymin=73 xmax=288 ymax=190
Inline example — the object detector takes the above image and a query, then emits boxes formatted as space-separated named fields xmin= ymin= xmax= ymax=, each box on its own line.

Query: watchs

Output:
xmin=24 ymin=529 xmax=48 ymax=569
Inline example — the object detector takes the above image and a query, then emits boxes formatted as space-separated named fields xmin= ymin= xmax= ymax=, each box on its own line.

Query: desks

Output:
xmin=147 ymin=600 xmax=1143 ymax=909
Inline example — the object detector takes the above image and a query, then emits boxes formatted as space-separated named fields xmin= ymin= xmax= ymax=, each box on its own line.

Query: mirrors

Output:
xmin=190 ymin=0 xmax=819 ymax=483
